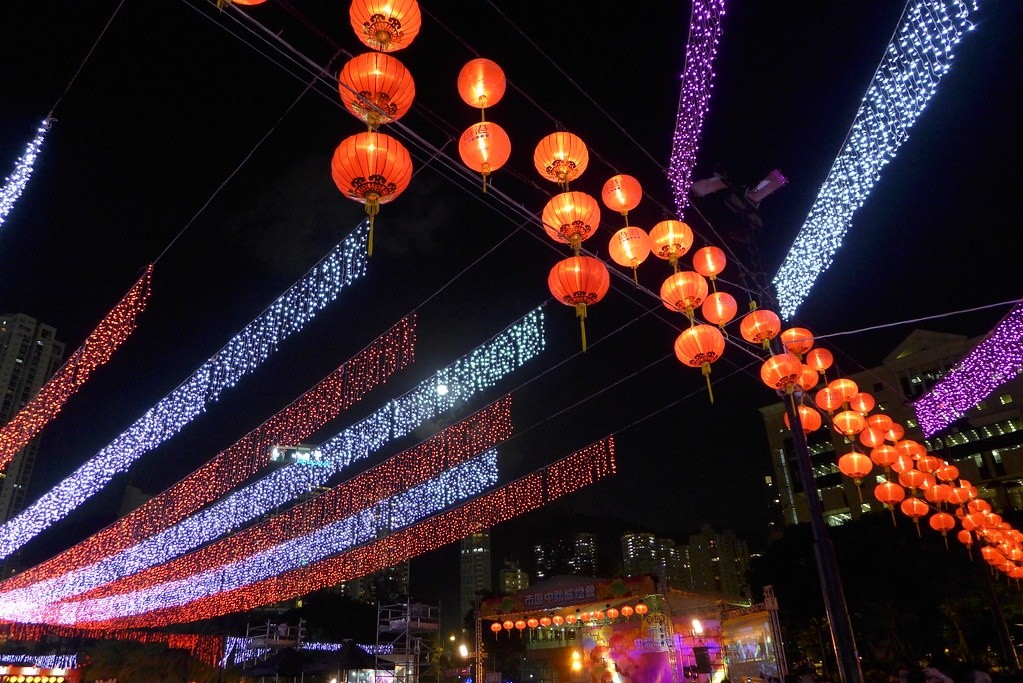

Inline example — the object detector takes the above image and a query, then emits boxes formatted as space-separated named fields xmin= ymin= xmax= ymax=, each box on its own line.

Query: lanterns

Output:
xmin=740 ymin=309 xmax=782 ymax=351
xmin=702 ymin=292 xmax=737 ymax=339
xmin=759 ymin=354 xmax=803 ymax=419
xmin=649 ymin=220 xmax=694 ymax=275
xmin=608 ymin=226 xmax=651 ymax=286
xmin=534 ymin=132 xmax=589 ymax=193
xmin=214 ymin=0 xmax=267 ymax=13
xmin=674 ymin=324 xmax=726 ymax=403
xmin=693 ymin=246 xmax=727 ymax=295
xmin=780 ymin=325 xmax=1023 ymax=583
xmin=331 ymin=132 xmax=414 ymax=257
xmin=456 ymin=58 xmax=506 ymax=122
xmin=348 ymin=0 xmax=422 ymax=52
xmin=338 ymin=52 xmax=416 ymax=133
xmin=491 ymin=604 xmax=650 ymax=642
xmin=541 ymin=190 xmax=600 ymax=256
xmin=459 ymin=122 xmax=511 ymax=193
xmin=548 ymin=255 xmax=611 ymax=353
xmin=601 ymin=175 xmax=643 ymax=228
xmin=659 ymin=270 xmax=708 ymax=329
